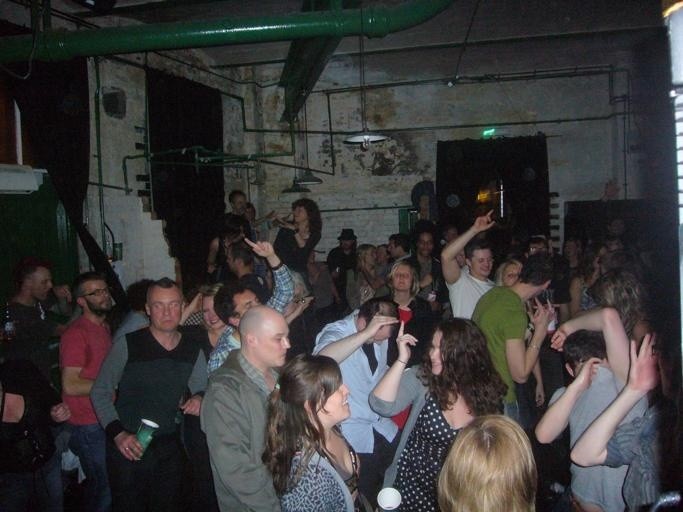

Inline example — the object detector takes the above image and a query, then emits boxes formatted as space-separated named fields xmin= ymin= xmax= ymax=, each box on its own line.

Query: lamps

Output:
xmin=345 ymin=35 xmax=389 ymax=150
xmin=0 ymin=100 xmax=48 ymax=195
xmin=295 ymin=102 xmax=323 ymax=185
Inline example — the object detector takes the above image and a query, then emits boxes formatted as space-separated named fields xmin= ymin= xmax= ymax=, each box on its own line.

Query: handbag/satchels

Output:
xmin=193 ymin=268 xmax=217 ymax=291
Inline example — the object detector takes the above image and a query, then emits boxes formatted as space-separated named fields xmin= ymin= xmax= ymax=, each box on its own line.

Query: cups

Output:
xmin=132 ymin=418 xmax=159 ymax=452
xmin=378 ymin=488 xmax=401 ymax=510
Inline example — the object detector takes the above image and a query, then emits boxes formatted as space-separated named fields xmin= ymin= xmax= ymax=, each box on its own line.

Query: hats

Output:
xmin=337 ymin=229 xmax=357 ymax=240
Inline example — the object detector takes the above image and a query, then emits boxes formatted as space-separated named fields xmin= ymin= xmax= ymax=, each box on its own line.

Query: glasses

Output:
xmin=78 ymin=287 xmax=114 ymax=296
xmin=526 ymin=247 xmax=547 ymax=252
xmin=416 ymin=240 xmax=434 ymax=245
xmin=651 ymin=343 xmax=678 ymax=357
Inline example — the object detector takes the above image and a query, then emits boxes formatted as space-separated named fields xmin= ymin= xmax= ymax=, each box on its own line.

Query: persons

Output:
xmin=1 ymin=189 xmax=683 ymax=512
